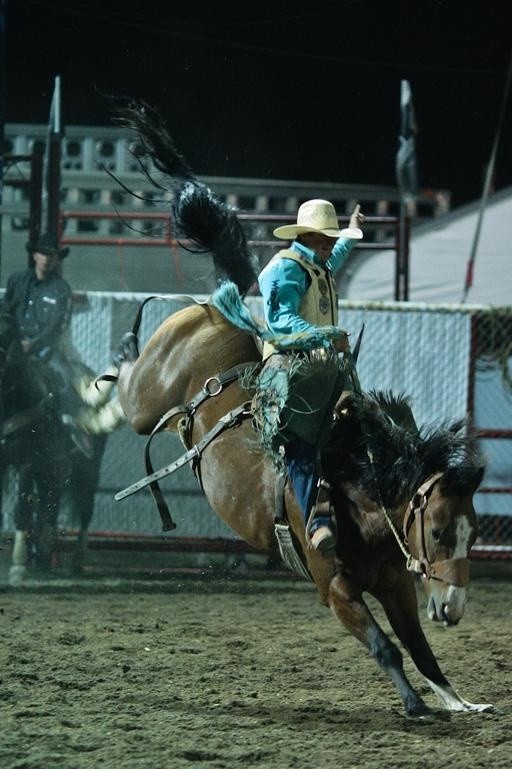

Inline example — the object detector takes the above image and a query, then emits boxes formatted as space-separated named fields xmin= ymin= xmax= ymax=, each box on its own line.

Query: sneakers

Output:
xmin=310 ymin=526 xmax=337 ymax=557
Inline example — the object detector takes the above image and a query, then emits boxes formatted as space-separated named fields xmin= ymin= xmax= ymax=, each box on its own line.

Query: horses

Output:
xmin=78 ymin=104 xmax=494 ymax=725
xmin=1 ymin=292 xmax=109 ymax=588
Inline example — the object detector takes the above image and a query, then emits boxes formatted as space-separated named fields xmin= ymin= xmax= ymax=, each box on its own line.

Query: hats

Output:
xmin=26 ymin=236 xmax=69 ymax=260
xmin=272 ymin=198 xmax=364 ymax=240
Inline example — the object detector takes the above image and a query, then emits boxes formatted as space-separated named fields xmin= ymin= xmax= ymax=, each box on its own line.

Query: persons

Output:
xmin=248 ymin=197 xmax=375 ymax=557
xmin=0 ymin=235 xmax=83 ymax=469
